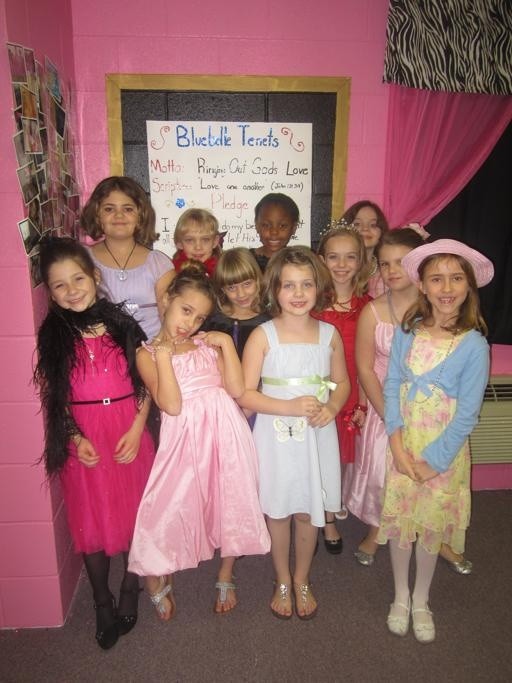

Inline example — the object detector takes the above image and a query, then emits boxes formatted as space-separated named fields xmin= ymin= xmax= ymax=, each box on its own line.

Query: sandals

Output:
xmin=412 ymin=601 xmax=436 ymax=643
xmin=356 ymin=539 xmax=377 ymax=566
xmin=439 ymin=550 xmax=472 ymax=575
xmin=293 ymin=577 xmax=317 ymax=620
xmin=214 ymin=580 xmax=237 ymax=614
xmin=151 ymin=574 xmax=177 ymax=622
xmin=270 ymin=581 xmax=293 ymax=620
xmin=387 ymin=597 xmax=413 ymax=637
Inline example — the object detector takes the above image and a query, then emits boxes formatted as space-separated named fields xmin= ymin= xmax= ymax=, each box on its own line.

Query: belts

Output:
xmin=67 ymin=392 xmax=137 ymax=405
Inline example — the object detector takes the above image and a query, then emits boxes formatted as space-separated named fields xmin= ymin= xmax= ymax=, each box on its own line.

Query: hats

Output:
xmin=402 ymin=239 xmax=494 ymax=289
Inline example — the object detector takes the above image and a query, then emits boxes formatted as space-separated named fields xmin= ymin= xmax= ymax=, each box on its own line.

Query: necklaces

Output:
xmin=383 ymin=289 xmax=412 ymax=343
xmin=145 ymin=326 xmax=191 ymax=347
xmin=103 ymin=238 xmax=138 ymax=280
xmin=404 ymin=323 xmax=456 ymax=403
xmin=72 ymin=323 xmax=105 ymax=377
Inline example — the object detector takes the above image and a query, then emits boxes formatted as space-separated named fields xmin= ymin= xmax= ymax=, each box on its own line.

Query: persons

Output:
xmin=204 ymin=248 xmax=261 ymax=360
xmin=308 ymin=220 xmax=380 ymax=556
xmin=168 ymin=203 xmax=226 ymax=277
xmin=380 ymin=234 xmax=489 ymax=640
xmin=243 ymin=191 xmax=299 ymax=268
xmin=337 ymin=201 xmax=386 ymax=298
xmin=80 ymin=173 xmax=175 ymax=329
xmin=243 ymin=245 xmax=349 ymax=615
xmin=132 ymin=266 xmax=251 ymax=624
xmin=354 ymin=227 xmax=473 ymax=576
xmin=34 ymin=238 xmax=157 ymax=649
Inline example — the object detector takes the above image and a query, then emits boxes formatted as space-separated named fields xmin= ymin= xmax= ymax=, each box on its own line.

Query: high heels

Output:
xmin=323 ymin=521 xmax=342 ymax=554
xmin=117 ymin=585 xmax=143 ymax=636
xmin=95 ymin=595 xmax=118 ymax=650
